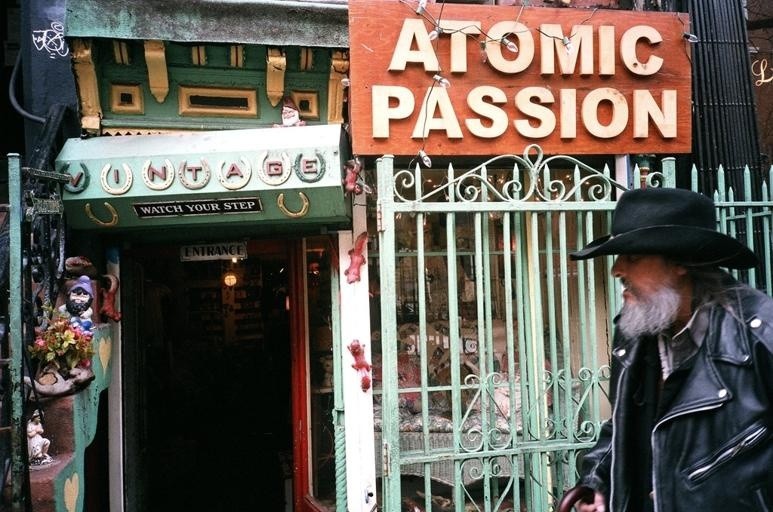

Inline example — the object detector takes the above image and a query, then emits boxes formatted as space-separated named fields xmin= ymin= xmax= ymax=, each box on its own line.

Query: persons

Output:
xmin=58 ymin=274 xmax=94 ymax=329
xmin=555 ymin=187 xmax=772 ymax=511
xmin=26 ymin=409 xmax=51 ymax=463
xmin=273 ymin=103 xmax=305 ymax=128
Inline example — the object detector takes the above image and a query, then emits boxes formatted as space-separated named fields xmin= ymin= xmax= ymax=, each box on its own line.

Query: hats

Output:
xmin=568 ymin=186 xmax=759 ymax=271
